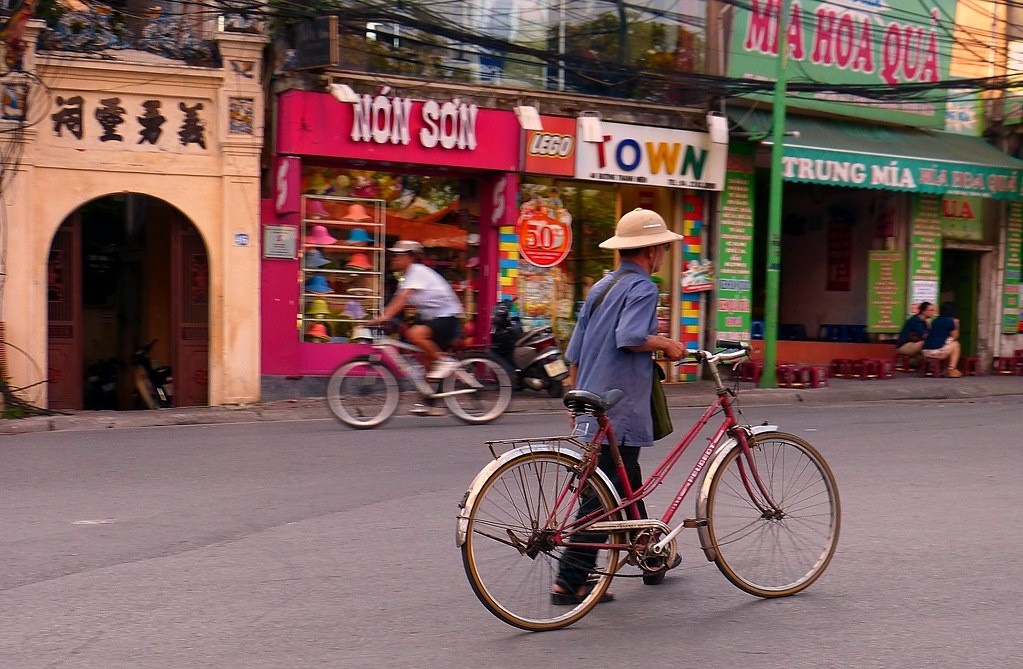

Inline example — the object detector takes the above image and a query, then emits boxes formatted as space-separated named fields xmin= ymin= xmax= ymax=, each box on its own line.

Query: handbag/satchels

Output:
xmin=648 ymin=362 xmax=674 ymax=441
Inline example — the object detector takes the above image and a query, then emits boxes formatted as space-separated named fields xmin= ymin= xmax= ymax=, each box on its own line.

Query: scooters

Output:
xmin=489 ymin=295 xmax=571 ymax=398
xmin=126 ymin=339 xmax=174 ymax=411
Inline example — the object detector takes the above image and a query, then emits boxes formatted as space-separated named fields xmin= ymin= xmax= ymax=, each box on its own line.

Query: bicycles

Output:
xmin=457 ymin=340 xmax=841 ymax=632
xmin=326 ymin=321 xmax=516 ymax=430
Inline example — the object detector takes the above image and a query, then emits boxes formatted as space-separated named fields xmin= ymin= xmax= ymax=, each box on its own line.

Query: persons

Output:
xmin=895 ymin=302 xmax=934 ymax=355
xmin=921 ymin=302 xmax=961 ymax=377
xmin=369 ymin=240 xmax=465 ymax=414
xmin=551 ymin=208 xmax=688 ymax=605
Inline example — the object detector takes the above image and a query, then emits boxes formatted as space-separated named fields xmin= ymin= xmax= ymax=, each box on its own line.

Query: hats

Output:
xmin=302 ymin=173 xmax=376 ymax=341
xmin=597 ymin=208 xmax=683 ymax=250
xmin=387 ymin=240 xmax=424 ymax=254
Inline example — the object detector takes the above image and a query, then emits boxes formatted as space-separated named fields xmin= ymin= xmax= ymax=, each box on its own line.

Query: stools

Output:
xmin=742 ymin=320 xmax=1023 ymax=389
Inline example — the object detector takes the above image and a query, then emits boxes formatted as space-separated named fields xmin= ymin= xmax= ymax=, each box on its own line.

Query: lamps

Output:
xmin=325 ymin=82 xmax=358 ymax=103
xmin=577 ymin=110 xmax=604 ymax=142
xmin=706 ymin=110 xmax=728 ymax=144
xmin=513 ymin=105 xmax=543 ymax=131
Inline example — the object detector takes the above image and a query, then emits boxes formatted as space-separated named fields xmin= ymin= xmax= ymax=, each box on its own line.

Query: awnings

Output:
xmin=727 ymin=106 xmax=1023 ymax=201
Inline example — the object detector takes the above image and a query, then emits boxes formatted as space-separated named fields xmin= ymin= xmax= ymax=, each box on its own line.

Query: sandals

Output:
xmin=550 ymin=578 xmax=613 ymax=604
xmin=642 ymin=553 xmax=682 ymax=585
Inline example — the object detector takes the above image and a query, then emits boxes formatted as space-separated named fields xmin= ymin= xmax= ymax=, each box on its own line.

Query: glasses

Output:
xmin=391 ymin=252 xmax=409 ymax=257
xmin=663 ymin=242 xmax=671 ymax=250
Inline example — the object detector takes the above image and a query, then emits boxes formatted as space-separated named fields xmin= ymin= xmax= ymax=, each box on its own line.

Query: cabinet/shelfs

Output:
xmin=300 ymin=193 xmax=387 ymax=345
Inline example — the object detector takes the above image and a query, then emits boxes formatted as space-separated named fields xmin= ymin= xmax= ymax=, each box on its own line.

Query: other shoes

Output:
xmin=408 ymin=406 xmax=443 ymax=416
xmin=947 ymin=370 xmax=961 ymax=377
xmin=425 ymin=358 xmax=463 ymax=379
